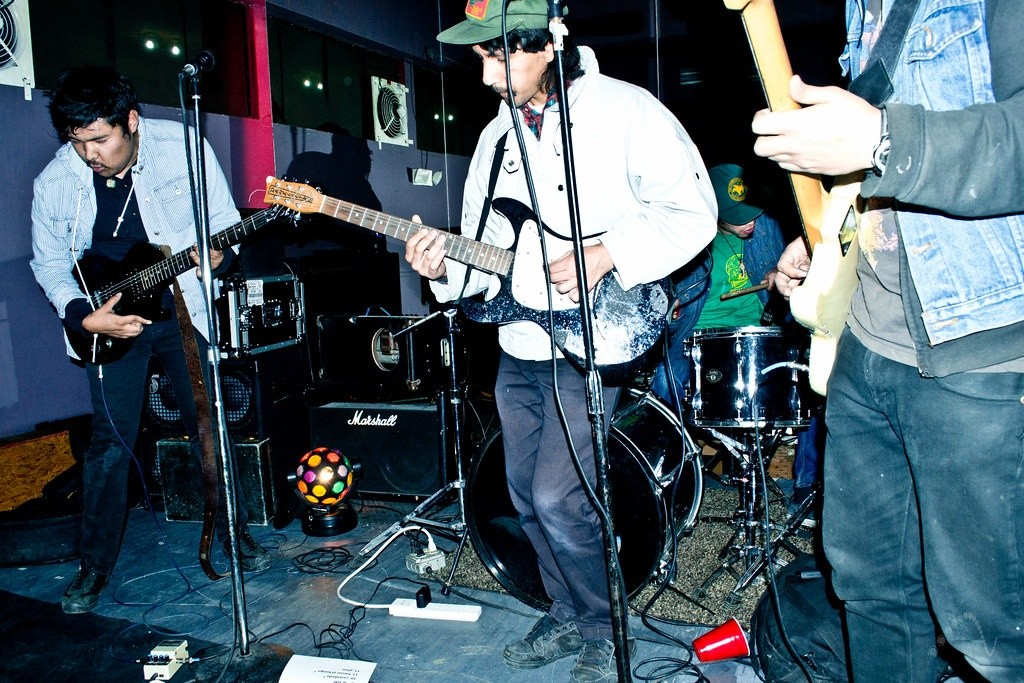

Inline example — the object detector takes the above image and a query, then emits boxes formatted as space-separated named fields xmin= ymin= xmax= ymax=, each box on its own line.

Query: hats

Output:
xmin=709 ymin=164 xmax=767 ymax=226
xmin=436 ymin=0 xmax=568 ymax=45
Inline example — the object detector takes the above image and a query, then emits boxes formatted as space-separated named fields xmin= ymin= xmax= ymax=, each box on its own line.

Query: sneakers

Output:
xmin=568 ymin=636 xmax=638 ymax=683
xmin=501 ymin=612 xmax=584 ymax=670
xmin=62 ymin=560 xmax=110 ymax=616
xmin=221 ymin=529 xmax=272 ymax=574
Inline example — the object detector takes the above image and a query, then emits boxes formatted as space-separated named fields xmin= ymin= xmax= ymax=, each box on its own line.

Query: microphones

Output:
xmin=178 ymin=49 xmax=216 ymax=78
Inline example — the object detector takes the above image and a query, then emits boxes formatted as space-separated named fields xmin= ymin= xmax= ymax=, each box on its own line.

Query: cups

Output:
xmin=692 ymin=615 xmax=750 ymax=664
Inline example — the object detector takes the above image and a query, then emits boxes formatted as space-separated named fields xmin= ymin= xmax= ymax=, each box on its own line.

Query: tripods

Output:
xmin=694 ymin=424 xmax=814 ymax=600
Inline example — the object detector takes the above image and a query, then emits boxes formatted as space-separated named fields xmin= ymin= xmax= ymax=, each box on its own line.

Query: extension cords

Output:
xmin=388 ymin=598 xmax=483 ymax=622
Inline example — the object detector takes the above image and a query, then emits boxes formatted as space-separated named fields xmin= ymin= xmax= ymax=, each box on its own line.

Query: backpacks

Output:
xmin=749 ymin=553 xmax=958 ymax=683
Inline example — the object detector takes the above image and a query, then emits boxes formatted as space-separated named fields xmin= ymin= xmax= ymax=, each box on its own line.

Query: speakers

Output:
xmin=144 ymin=340 xmax=314 ymax=444
xmin=318 ymin=400 xmax=461 ymax=504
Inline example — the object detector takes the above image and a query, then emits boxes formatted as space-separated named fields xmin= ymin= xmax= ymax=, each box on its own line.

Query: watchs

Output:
xmin=873 ymin=108 xmax=891 ymax=176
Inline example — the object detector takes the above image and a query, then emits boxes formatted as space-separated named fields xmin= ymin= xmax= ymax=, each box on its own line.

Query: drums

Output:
xmin=462 ymin=388 xmax=705 ymax=615
xmin=686 ymin=323 xmax=815 ymax=430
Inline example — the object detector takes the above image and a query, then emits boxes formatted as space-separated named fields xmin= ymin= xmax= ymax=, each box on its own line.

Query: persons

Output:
xmin=651 ymin=164 xmax=830 ymax=526
xmin=29 ymin=64 xmax=246 ymax=615
xmin=405 ymin=0 xmax=720 ymax=683
xmin=752 ymin=0 xmax=1024 ymax=683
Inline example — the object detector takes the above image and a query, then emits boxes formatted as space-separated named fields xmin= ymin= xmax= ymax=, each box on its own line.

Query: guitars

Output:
xmin=63 ymin=201 xmax=303 ymax=366
xmin=724 ymin=0 xmax=864 ymax=395
xmin=264 ymin=172 xmax=672 ymax=368
